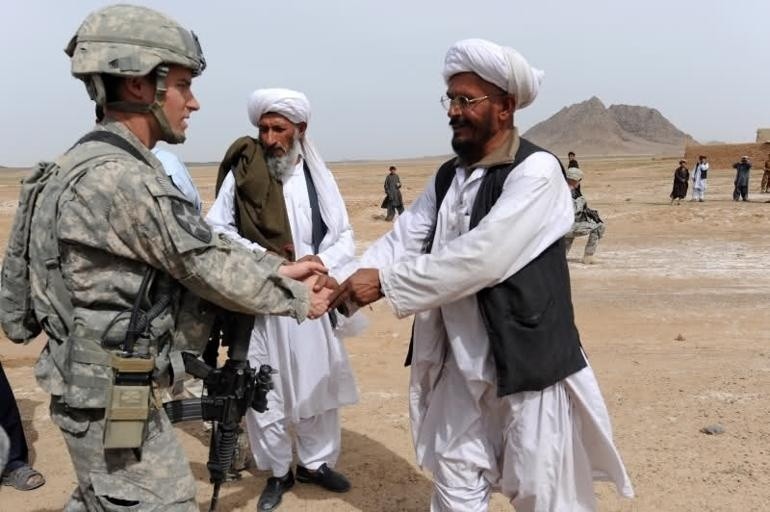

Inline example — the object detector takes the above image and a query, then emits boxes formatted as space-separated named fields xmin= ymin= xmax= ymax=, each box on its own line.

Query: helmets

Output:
xmin=567 ymin=166 xmax=584 ymax=180
xmin=69 ymin=4 xmax=206 ymax=78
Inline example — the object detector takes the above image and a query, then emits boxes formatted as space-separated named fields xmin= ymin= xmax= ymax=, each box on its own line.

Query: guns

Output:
xmin=163 ymin=313 xmax=274 ymax=512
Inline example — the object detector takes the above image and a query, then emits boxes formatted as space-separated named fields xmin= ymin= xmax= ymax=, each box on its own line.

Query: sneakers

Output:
xmin=582 ymin=255 xmax=601 ymax=264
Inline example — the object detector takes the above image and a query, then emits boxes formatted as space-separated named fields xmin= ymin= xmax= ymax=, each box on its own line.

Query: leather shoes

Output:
xmin=257 ymin=467 xmax=295 ymax=510
xmin=295 ymin=464 xmax=350 ymax=493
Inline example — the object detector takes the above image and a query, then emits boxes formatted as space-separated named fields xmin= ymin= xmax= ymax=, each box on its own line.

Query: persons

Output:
xmin=567 ymin=152 xmax=581 ymax=196
xmin=0 ymin=1 xmax=335 ymax=511
xmin=0 ymin=362 xmax=46 ymax=492
xmin=688 ymin=153 xmax=710 ymax=203
xmin=199 ymin=87 xmax=363 ymax=512
xmin=380 ymin=166 xmax=404 ymax=221
xmin=732 ymin=154 xmax=752 ymax=202
xmin=310 ymin=39 xmax=639 ymax=511
xmin=561 ymin=166 xmax=608 ymax=265
xmin=760 ymin=152 xmax=770 ymax=193
xmin=668 ymin=158 xmax=691 ymax=206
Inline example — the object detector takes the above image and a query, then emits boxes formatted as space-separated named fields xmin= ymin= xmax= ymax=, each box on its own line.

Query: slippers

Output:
xmin=3 ymin=465 xmax=45 ymax=491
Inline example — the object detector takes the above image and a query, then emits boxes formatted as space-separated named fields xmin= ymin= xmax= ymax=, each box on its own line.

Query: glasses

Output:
xmin=439 ymin=90 xmax=504 ymax=109
